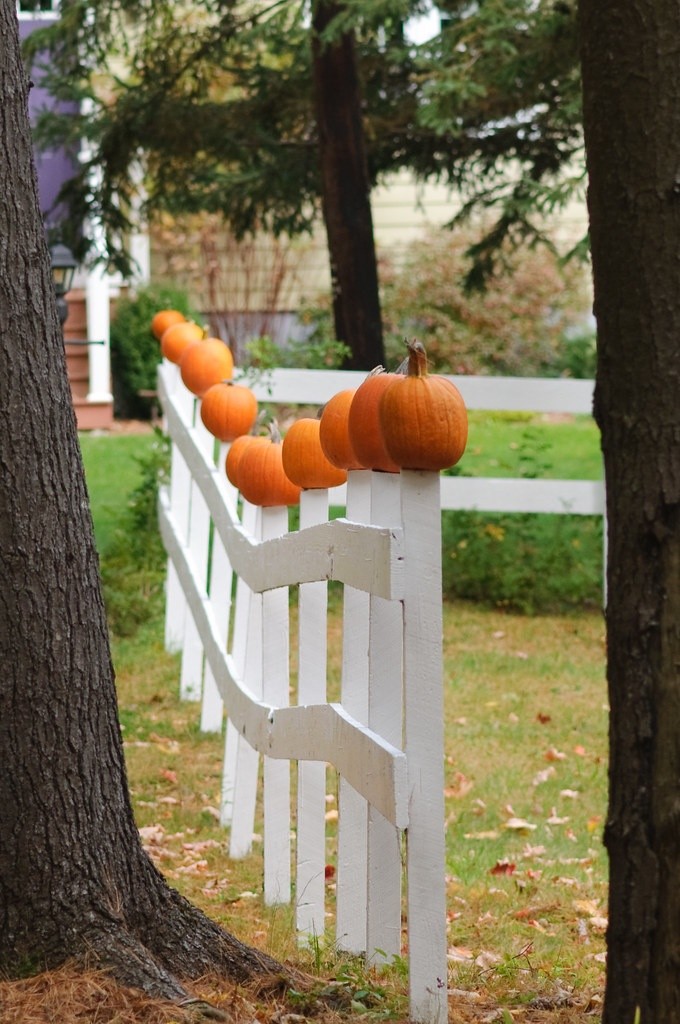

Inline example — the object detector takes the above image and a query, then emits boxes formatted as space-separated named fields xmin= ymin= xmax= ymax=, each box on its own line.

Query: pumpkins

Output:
xmin=200 ymin=380 xmax=259 ymax=441
xmin=152 ymin=309 xmax=233 ymax=398
xmin=225 ymin=339 xmax=469 ymax=507
